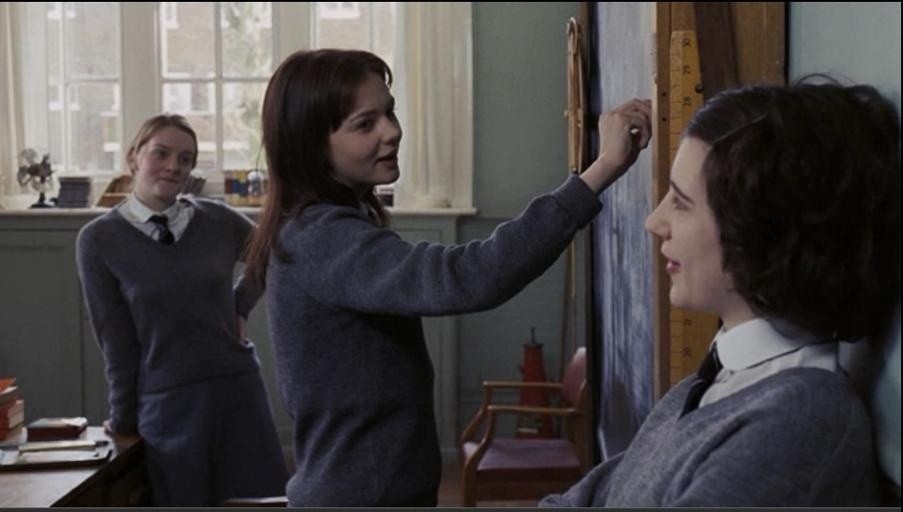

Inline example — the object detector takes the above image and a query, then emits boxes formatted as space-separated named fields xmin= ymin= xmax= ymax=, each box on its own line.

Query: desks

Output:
xmin=0 ymin=425 xmax=144 ymax=507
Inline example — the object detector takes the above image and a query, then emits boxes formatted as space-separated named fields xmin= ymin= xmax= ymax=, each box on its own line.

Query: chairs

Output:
xmin=459 ymin=346 xmax=592 ymax=507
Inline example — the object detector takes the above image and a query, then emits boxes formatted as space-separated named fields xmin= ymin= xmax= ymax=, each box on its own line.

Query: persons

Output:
xmin=76 ymin=111 xmax=291 ymax=507
xmin=533 ymin=72 xmax=900 ymax=509
xmin=235 ymin=48 xmax=653 ymax=508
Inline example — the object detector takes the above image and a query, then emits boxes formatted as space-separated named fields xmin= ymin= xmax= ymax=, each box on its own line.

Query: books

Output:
xmin=0 ymin=378 xmax=25 ymax=442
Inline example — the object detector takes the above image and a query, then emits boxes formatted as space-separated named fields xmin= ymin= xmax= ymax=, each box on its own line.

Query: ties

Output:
xmin=150 ymin=215 xmax=174 ymax=244
xmin=680 ymin=342 xmax=723 ymax=414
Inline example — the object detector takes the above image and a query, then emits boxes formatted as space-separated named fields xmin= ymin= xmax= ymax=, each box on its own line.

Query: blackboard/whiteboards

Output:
xmin=595 ymin=2 xmax=652 ymax=460
